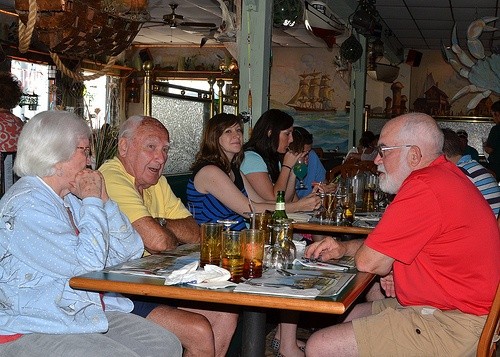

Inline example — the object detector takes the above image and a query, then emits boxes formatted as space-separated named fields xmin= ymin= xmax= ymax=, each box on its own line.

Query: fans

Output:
xmin=146 ymin=5 xmax=216 ymax=28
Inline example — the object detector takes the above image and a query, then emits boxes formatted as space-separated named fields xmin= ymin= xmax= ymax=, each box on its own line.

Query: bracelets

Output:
xmin=281 ymin=165 xmax=292 ymax=171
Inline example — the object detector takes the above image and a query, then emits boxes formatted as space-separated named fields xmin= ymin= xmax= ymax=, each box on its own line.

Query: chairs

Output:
xmin=476 ymin=281 xmax=500 ymax=357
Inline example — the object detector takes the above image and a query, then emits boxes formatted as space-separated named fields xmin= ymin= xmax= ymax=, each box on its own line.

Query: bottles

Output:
xmin=271 ymin=190 xmax=289 ymax=243
xmin=316 ymin=193 xmax=346 ymax=213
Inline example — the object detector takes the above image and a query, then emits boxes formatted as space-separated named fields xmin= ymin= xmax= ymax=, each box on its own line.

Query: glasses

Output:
xmin=77 ymin=147 xmax=91 ymax=156
xmin=377 ymin=145 xmax=422 ymax=157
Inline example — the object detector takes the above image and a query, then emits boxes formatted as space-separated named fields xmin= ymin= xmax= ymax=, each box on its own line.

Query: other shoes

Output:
xmin=273 ymin=350 xmax=286 ymax=357
xmin=271 ymin=337 xmax=305 ymax=352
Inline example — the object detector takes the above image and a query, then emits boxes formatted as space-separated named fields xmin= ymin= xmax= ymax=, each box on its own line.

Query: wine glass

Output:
xmin=217 ymin=219 xmax=238 ymax=232
xmin=262 ymin=218 xmax=296 ymax=272
xmin=293 ymin=157 xmax=307 ymax=189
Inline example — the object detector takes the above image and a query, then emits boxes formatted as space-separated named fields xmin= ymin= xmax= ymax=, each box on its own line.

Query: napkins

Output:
xmin=287 ymin=212 xmax=312 ymax=226
xmin=165 ymin=262 xmax=233 ymax=291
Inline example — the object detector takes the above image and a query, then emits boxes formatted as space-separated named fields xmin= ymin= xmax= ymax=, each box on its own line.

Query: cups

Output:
xmin=200 ymin=222 xmax=223 ymax=269
xmin=342 ymin=173 xmax=376 ymax=222
xmin=242 ymin=229 xmax=265 ymax=278
xmin=251 ymin=213 xmax=271 ymax=245
xmin=222 ymin=231 xmax=245 ymax=283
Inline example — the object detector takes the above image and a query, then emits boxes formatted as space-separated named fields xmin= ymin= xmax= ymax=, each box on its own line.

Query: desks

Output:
xmin=290 ymin=199 xmax=389 ymax=241
xmin=69 ymin=237 xmax=378 ymax=356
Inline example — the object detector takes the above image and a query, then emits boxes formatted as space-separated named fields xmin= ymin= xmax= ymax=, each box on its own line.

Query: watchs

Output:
xmin=155 ymin=217 xmax=167 ymax=228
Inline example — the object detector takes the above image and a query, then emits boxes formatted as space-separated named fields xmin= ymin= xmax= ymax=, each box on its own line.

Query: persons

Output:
xmin=304 ymin=112 xmax=500 ymax=357
xmin=232 ymin=108 xmax=500 ymax=220
xmin=482 ymin=100 xmax=500 ymax=182
xmin=184 ymin=113 xmax=321 ymax=357
xmin=0 ymin=109 xmax=183 ymax=357
xmin=0 ymin=70 xmax=25 ymax=155
xmin=97 ymin=114 xmax=238 ymax=357
